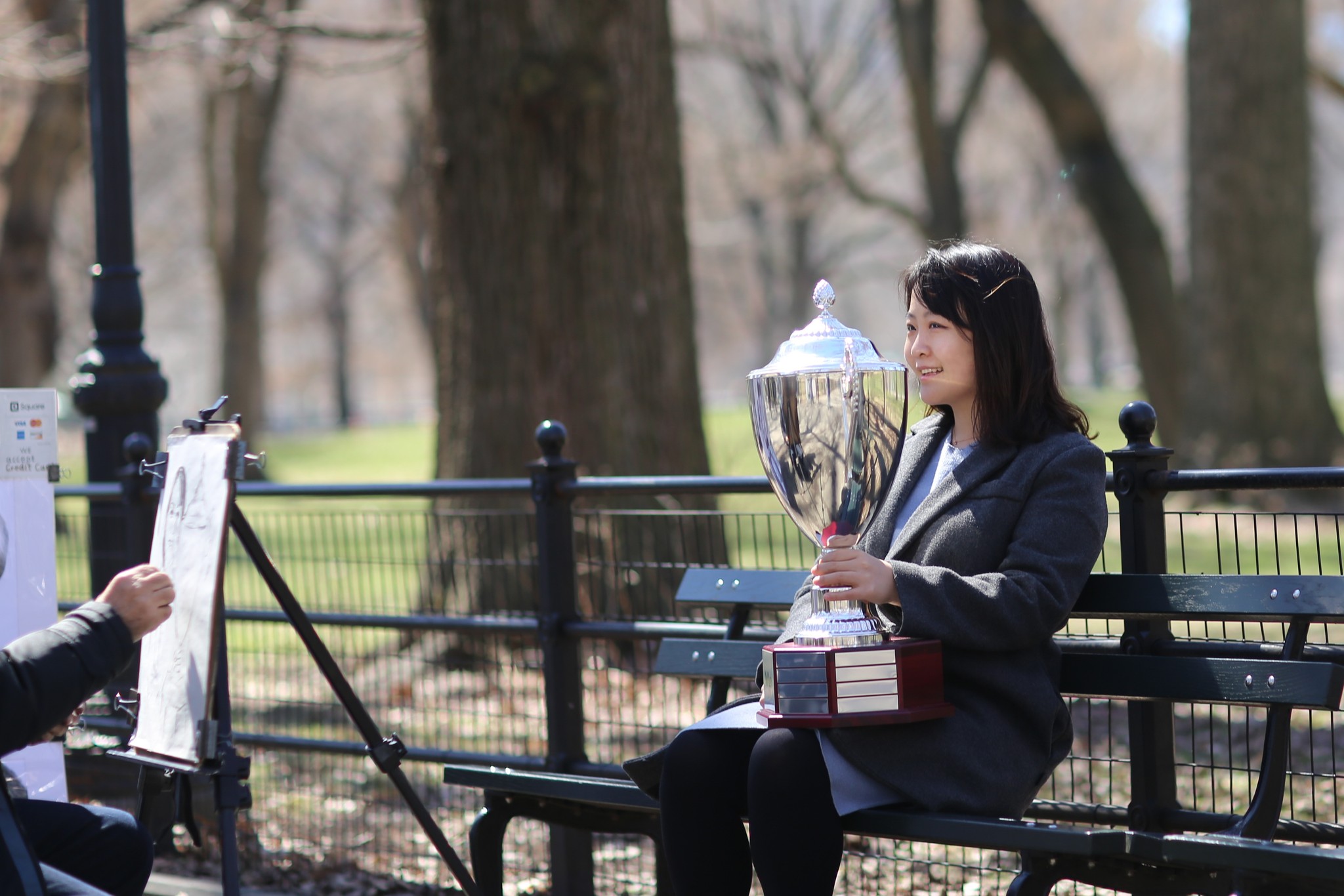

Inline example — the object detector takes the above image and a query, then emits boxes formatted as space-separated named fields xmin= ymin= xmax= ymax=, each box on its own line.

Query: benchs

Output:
xmin=443 ymin=566 xmax=1344 ymax=896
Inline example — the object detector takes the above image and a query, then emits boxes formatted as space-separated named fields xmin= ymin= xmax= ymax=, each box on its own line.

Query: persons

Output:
xmin=622 ymin=243 xmax=1110 ymax=896
xmin=0 ymin=564 xmax=176 ymax=896
xmin=778 ymin=376 xmax=805 ymax=476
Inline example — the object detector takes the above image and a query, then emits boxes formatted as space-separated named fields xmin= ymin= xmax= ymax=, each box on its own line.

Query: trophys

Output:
xmin=747 ymin=280 xmax=956 ymax=729
